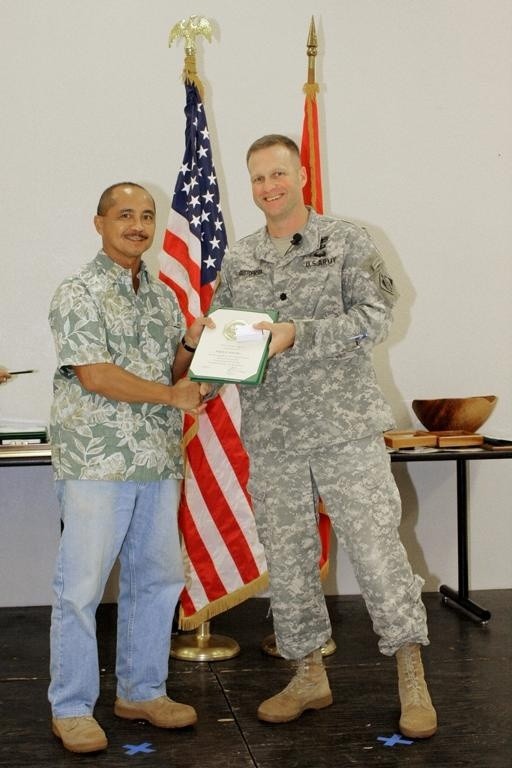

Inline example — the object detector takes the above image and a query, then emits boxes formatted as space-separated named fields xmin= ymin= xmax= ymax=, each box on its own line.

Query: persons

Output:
xmin=190 ymin=133 xmax=438 ymax=738
xmin=0 ymin=365 xmax=13 ymax=384
xmin=45 ymin=179 xmax=216 ymax=755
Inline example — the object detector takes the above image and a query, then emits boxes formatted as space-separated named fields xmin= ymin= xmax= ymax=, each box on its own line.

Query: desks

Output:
xmin=0 ymin=433 xmax=510 ymax=625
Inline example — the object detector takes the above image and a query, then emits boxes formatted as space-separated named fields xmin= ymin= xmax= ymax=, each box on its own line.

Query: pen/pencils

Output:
xmin=8 ymin=370 xmax=35 ymax=374
xmin=347 ymin=334 xmax=368 ymax=341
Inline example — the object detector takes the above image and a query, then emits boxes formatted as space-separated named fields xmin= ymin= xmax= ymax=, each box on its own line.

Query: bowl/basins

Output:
xmin=410 ymin=395 xmax=501 ymax=430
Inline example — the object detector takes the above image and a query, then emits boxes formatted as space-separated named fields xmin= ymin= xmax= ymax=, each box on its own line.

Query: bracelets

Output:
xmin=181 ymin=336 xmax=196 ymax=353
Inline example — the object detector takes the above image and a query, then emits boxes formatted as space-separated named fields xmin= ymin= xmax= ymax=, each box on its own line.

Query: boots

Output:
xmin=395 ymin=638 xmax=439 ymax=740
xmin=256 ymin=646 xmax=334 ymax=724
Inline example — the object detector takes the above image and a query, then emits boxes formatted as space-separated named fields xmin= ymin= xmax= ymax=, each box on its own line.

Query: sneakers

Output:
xmin=49 ymin=713 xmax=109 ymax=755
xmin=113 ymin=693 xmax=199 ymax=729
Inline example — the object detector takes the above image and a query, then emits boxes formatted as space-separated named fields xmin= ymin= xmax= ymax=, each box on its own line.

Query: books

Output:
xmin=0 ymin=419 xmax=49 ymax=445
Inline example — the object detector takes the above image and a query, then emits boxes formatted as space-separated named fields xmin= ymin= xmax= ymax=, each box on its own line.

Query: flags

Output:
xmin=159 ymin=81 xmax=334 ymax=633
xmin=295 ymin=91 xmax=333 ymax=584
